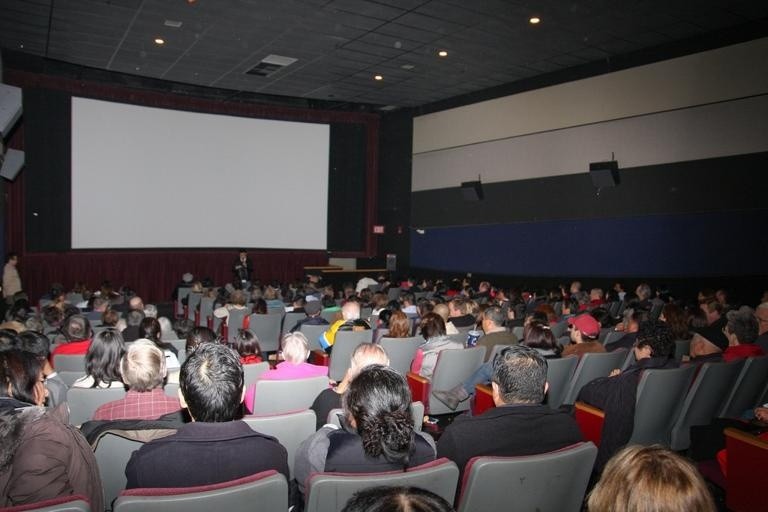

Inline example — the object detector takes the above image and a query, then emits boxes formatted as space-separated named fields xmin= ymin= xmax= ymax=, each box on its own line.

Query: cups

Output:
xmin=468 ymin=331 xmax=479 ymax=349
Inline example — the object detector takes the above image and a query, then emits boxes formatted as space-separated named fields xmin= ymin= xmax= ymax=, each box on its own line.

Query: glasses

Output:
xmin=755 ymin=316 xmax=767 ymax=324
xmin=34 ymin=377 xmax=48 ymax=388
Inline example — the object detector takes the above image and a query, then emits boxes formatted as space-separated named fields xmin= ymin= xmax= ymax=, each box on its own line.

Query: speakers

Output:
xmin=460 ymin=180 xmax=485 ymax=203
xmin=588 ymin=159 xmax=621 ymax=189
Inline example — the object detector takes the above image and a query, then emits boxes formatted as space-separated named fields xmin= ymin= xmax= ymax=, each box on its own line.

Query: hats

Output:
xmin=567 ymin=313 xmax=601 ymax=339
xmin=689 ymin=325 xmax=730 ymax=351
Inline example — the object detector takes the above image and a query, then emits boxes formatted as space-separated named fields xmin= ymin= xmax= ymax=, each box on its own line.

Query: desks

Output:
xmin=322 ymin=269 xmax=390 ymax=282
xmin=303 ymin=266 xmax=343 ymax=279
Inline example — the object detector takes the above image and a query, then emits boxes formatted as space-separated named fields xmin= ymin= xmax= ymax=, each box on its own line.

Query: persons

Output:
xmin=1 ymin=251 xmax=768 ymax=511
